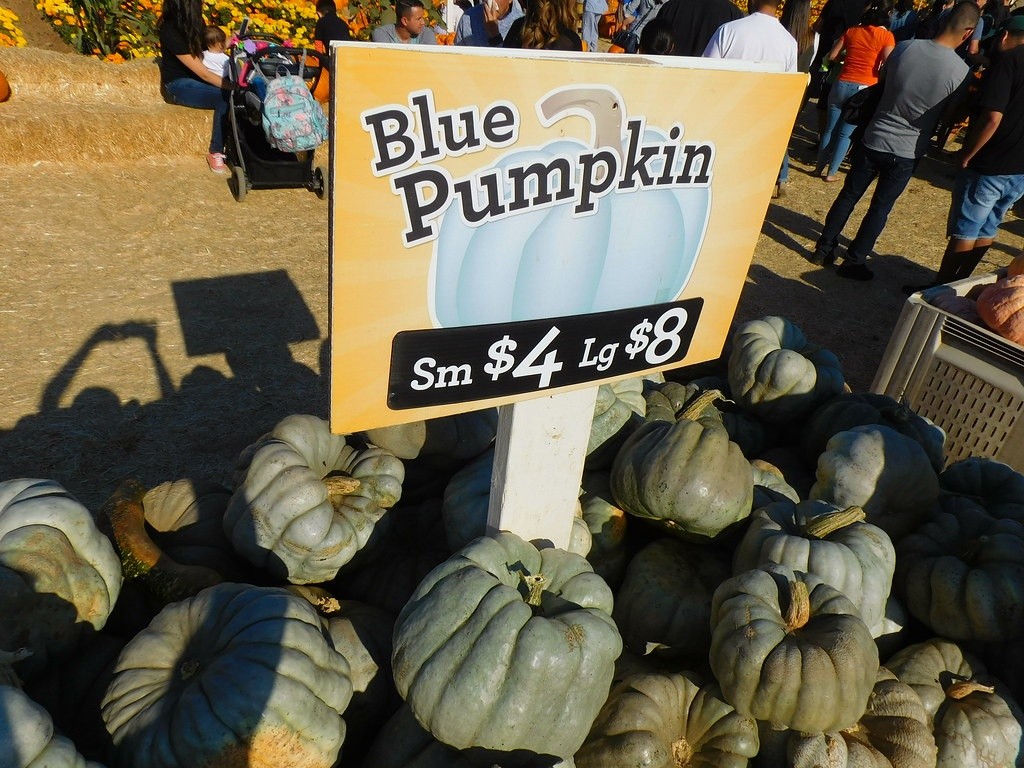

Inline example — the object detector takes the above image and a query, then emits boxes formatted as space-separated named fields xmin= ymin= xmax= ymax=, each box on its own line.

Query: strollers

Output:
xmin=225 ymin=32 xmax=331 ymax=203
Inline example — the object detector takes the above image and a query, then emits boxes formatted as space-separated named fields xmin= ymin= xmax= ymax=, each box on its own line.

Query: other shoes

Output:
xmin=838 ymin=262 xmax=875 ymax=281
xmin=825 ymin=175 xmax=840 ymax=181
xmin=778 ymin=188 xmax=789 ymax=197
xmin=811 ymin=250 xmax=836 ymax=265
xmin=902 ymin=280 xmax=943 ymax=295
xmin=808 ymin=170 xmax=821 ymax=177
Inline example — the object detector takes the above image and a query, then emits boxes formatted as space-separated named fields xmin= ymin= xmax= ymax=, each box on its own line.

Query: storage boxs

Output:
xmin=870 ymin=272 xmax=1024 ymax=481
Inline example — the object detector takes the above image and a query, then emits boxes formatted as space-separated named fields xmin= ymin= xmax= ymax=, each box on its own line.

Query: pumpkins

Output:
xmin=435 ymin=30 xmax=456 ymax=45
xmin=598 ymin=0 xmax=623 ymax=37
xmin=0 ymin=313 xmax=1024 ymax=768
xmin=975 ymin=252 xmax=1024 ymax=346
xmin=608 ymin=45 xmax=625 ymax=53
xmin=298 ymin=55 xmax=330 ymax=105
xmin=0 ymin=70 xmax=9 ymax=102
xmin=582 ymin=40 xmax=587 ymax=51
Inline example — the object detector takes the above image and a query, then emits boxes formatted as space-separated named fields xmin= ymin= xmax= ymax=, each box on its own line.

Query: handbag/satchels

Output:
xmin=842 ymin=79 xmax=884 ymax=125
xmin=612 ymin=28 xmax=640 ymax=54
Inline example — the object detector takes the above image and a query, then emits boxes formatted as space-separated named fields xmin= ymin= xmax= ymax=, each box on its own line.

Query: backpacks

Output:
xmin=250 ymin=48 xmax=329 ymax=152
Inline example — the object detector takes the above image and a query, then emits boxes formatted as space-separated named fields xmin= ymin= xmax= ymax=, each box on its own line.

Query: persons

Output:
xmin=373 ymin=0 xmax=437 ymax=45
xmin=455 ymin=0 xmax=822 ymax=198
xmin=811 ymin=0 xmax=1024 ymax=181
xmin=901 ymin=7 xmax=1024 ymax=297
xmin=158 ymin=0 xmax=234 ymax=174
xmin=813 ymin=2 xmax=980 ymax=280
xmin=314 ymin=0 xmax=350 ymax=54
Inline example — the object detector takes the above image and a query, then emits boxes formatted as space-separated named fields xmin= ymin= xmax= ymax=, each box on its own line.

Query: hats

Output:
xmin=1006 ymin=15 xmax=1024 ymax=30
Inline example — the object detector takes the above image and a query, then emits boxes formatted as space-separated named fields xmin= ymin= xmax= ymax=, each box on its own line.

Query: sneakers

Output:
xmin=207 ymin=152 xmax=226 ymax=174
